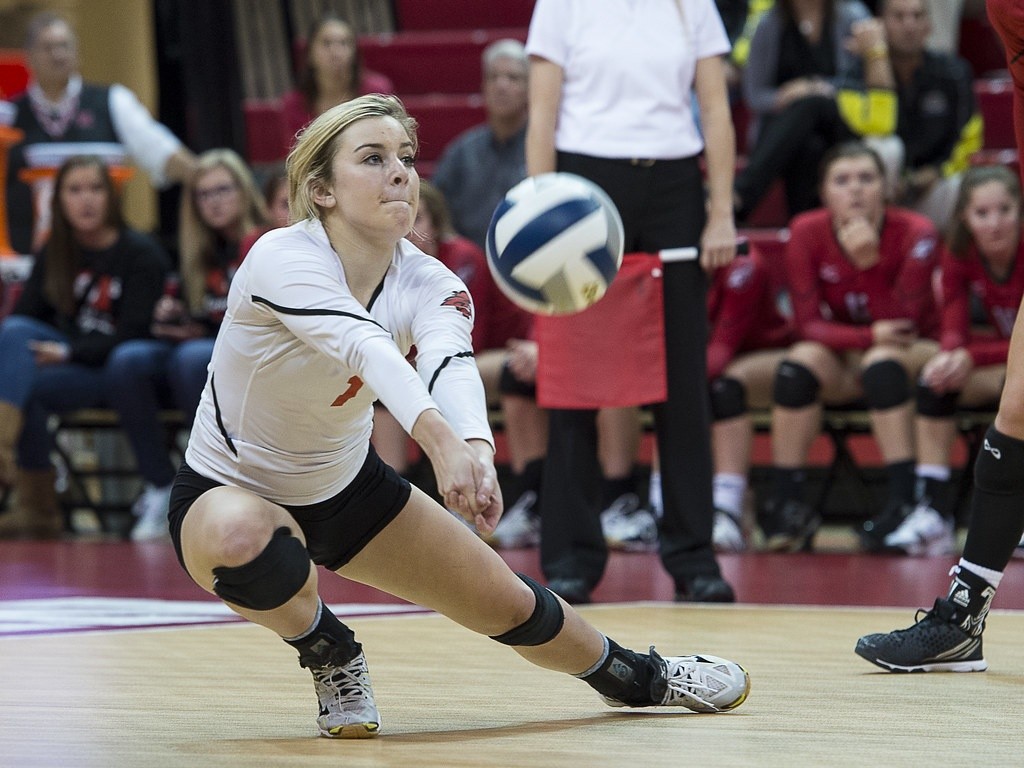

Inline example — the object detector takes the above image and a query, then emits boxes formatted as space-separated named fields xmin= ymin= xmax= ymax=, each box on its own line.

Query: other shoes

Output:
xmin=128 ymin=481 xmax=173 ymax=540
xmin=882 ymin=494 xmax=957 ymax=556
xmin=486 ymin=490 xmax=540 ymax=549
xmin=710 ymin=507 xmax=765 ymax=555
xmin=599 ymin=491 xmax=660 ymax=554
xmin=546 ymin=573 xmax=593 ymax=604
xmin=852 ymin=506 xmax=915 ymax=554
xmin=764 ymin=495 xmax=824 ymax=557
xmin=674 ymin=574 xmax=735 ymax=602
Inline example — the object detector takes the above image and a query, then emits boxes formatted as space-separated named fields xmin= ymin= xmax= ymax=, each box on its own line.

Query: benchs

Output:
xmin=243 ymin=0 xmax=1024 ymax=278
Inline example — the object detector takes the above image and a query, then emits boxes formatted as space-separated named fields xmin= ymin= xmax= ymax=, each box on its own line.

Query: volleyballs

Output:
xmin=485 ymin=171 xmax=625 ymax=318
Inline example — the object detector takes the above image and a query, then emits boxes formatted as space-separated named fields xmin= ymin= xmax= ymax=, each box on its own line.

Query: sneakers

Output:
xmin=308 ymin=640 xmax=381 ymax=739
xmin=855 ymin=595 xmax=988 ymax=673
xmin=598 ymin=645 xmax=750 ymax=713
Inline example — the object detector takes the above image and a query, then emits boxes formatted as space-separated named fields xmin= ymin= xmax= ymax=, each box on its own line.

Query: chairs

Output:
xmin=23 ymin=260 xmax=977 ymax=555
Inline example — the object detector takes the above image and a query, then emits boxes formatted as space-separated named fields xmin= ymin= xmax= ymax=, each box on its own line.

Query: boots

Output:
xmin=0 ymin=464 xmax=65 ymax=540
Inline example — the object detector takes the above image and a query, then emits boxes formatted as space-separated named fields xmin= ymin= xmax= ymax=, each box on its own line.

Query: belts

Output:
xmin=585 ymin=156 xmax=670 ymax=168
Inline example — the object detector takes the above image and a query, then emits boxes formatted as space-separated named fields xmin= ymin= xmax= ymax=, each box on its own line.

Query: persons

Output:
xmin=762 ymin=143 xmax=945 ymax=551
xmin=855 ymin=291 xmax=1024 ymax=672
xmin=168 ymin=92 xmax=749 ymax=739
xmin=109 ymin=149 xmax=273 ymax=545
xmin=0 ymin=154 xmax=178 ymax=541
xmin=524 ymin=0 xmax=733 ymax=611
xmin=282 ymin=14 xmax=392 ymax=161
xmin=0 ymin=10 xmax=197 ymax=250
xmin=886 ymin=0 xmax=1024 ymax=557
xmin=603 ymin=174 xmax=752 ymax=553
xmin=490 ymin=309 xmax=635 ymax=554
xmin=431 ymin=38 xmax=559 ymax=247
xmin=688 ymin=0 xmax=983 ymax=229
xmin=260 ymin=166 xmax=294 ymax=227
xmin=371 ymin=180 xmax=533 ymax=540
